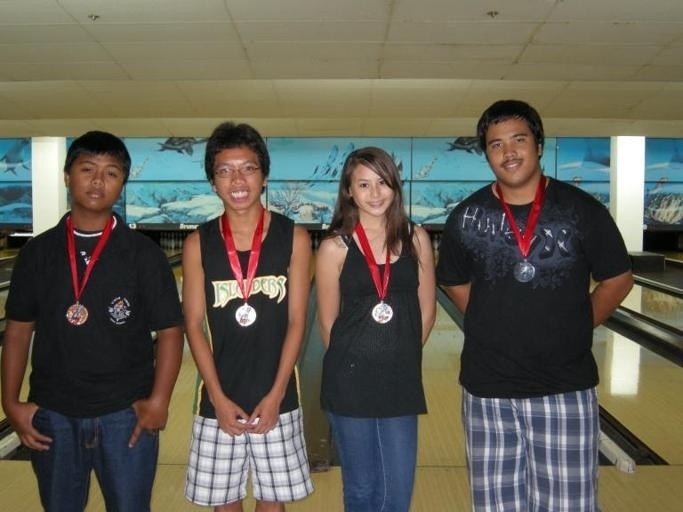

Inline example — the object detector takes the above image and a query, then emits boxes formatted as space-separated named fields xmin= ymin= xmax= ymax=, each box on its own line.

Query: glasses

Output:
xmin=214 ymin=166 xmax=261 ymax=179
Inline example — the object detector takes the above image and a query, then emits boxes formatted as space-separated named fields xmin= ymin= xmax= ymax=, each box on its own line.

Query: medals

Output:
xmin=514 ymin=259 xmax=537 ymax=285
xmin=64 ymin=302 xmax=88 ymax=326
xmin=231 ymin=303 xmax=260 ymax=328
xmin=370 ymin=302 xmax=397 ymax=323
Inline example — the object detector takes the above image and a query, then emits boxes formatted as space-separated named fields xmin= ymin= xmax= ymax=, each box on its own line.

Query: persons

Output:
xmin=1 ymin=131 xmax=185 ymax=512
xmin=314 ymin=145 xmax=436 ymax=512
xmin=179 ymin=122 xmax=312 ymax=512
xmin=439 ymin=100 xmax=636 ymax=512
